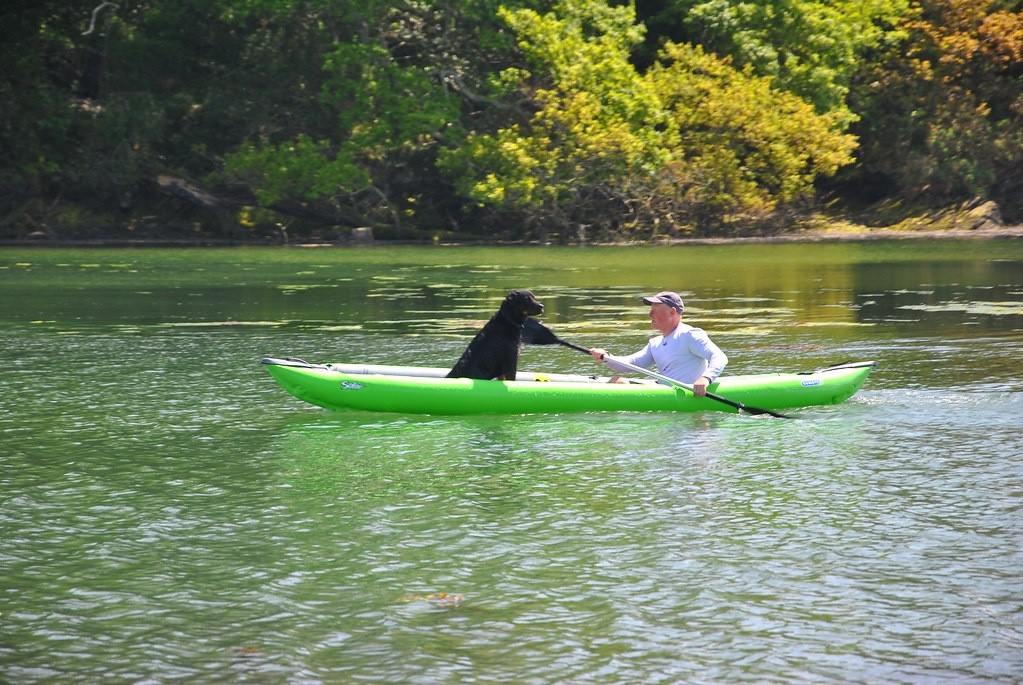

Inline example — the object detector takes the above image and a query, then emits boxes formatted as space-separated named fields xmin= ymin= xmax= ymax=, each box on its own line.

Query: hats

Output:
xmin=643 ymin=291 xmax=684 ymax=314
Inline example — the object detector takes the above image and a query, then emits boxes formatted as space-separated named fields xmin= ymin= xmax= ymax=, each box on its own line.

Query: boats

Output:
xmin=261 ymin=356 xmax=876 ymax=415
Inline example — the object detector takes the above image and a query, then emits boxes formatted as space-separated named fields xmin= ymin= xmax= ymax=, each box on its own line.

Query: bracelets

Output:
xmin=600 ymin=351 xmax=610 ymax=362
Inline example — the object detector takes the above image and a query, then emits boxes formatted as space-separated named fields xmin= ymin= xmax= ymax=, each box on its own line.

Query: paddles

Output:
xmin=519 ymin=315 xmax=790 ymax=419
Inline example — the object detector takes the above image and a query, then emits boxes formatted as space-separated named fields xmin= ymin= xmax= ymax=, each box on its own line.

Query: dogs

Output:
xmin=444 ymin=289 xmax=545 ymax=380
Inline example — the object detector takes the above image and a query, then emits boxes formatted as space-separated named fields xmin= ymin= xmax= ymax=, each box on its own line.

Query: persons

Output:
xmin=589 ymin=291 xmax=728 ymax=397
